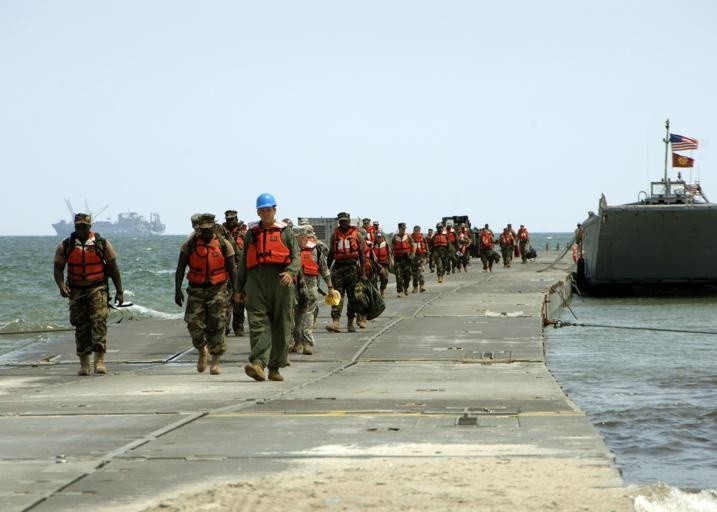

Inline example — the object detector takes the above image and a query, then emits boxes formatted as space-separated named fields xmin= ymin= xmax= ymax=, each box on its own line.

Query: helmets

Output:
xmin=255 ymin=192 xmax=277 ymax=209
xmin=325 ymin=289 xmax=342 ymax=307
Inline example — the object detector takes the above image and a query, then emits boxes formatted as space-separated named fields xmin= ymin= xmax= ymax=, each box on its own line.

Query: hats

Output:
xmin=398 ymin=222 xmax=408 ymax=229
xmin=413 ymin=221 xmax=526 ymax=232
xmin=362 ymin=218 xmax=385 ymax=238
xmin=73 ymin=212 xmax=92 ymax=226
xmin=195 ymin=213 xmax=217 ymax=230
xmin=337 ymin=212 xmax=350 ymax=221
xmin=190 ymin=213 xmax=202 ymax=229
xmin=224 ymin=209 xmax=237 ymax=219
xmin=293 ymin=224 xmax=315 ymax=237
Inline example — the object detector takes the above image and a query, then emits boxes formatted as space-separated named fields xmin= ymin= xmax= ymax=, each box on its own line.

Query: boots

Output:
xmin=196 ymin=345 xmax=210 ymax=373
xmin=93 ymin=351 xmax=108 ymax=374
xmin=396 ymin=257 xmax=529 ymax=300
xmin=325 ymin=318 xmax=341 ymax=333
xmin=378 ymin=288 xmax=387 ymax=299
xmin=77 ymin=354 xmax=92 ymax=376
xmin=358 ymin=320 xmax=368 ymax=329
xmin=346 ymin=317 xmax=356 ymax=332
xmin=267 ymin=367 xmax=285 ymax=381
xmin=291 ymin=342 xmax=313 ymax=355
xmin=244 ymin=358 xmax=266 ymax=381
xmin=208 ymin=352 xmax=222 ymax=375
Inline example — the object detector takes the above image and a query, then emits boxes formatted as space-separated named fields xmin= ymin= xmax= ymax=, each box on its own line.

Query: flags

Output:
xmin=672 ymin=152 xmax=695 ymax=167
xmin=670 ymin=132 xmax=698 ymax=151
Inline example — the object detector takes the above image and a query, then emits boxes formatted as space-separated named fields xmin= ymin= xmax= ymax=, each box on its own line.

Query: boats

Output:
xmin=578 ymin=118 xmax=716 ymax=294
xmin=52 ymin=198 xmax=166 ymax=236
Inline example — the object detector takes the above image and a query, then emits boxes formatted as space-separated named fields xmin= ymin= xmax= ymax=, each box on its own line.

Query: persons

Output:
xmin=573 ymin=222 xmax=584 ymax=245
xmin=52 ymin=211 xmax=124 ymax=375
